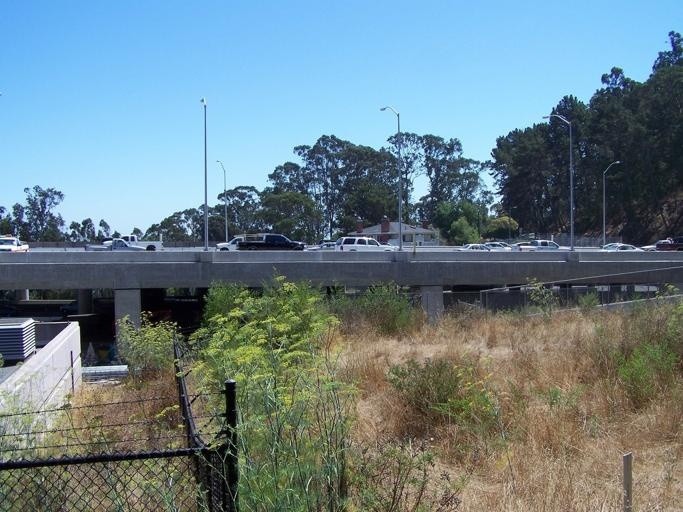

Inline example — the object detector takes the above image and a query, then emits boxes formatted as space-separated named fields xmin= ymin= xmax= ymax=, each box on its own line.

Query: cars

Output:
xmin=604 ymin=236 xmax=683 ymax=252
xmin=453 ymin=238 xmax=561 ymax=252
xmin=0 ymin=235 xmax=29 ymax=253
xmin=216 ymin=232 xmax=403 ymax=254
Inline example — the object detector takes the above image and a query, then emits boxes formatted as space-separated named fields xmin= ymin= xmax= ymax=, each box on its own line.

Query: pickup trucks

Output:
xmin=104 ymin=235 xmax=164 ymax=252
xmin=84 ymin=239 xmax=145 ymax=251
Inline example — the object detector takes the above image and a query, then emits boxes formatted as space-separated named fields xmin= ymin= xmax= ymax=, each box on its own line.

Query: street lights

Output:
xmin=601 ymin=160 xmax=622 ymax=247
xmin=509 ymin=205 xmax=518 ymax=240
xmin=381 ymin=105 xmax=404 ymax=251
xmin=201 ymin=97 xmax=209 ymax=251
xmin=216 ymin=159 xmax=230 ymax=241
xmin=543 ymin=114 xmax=574 ymax=250
